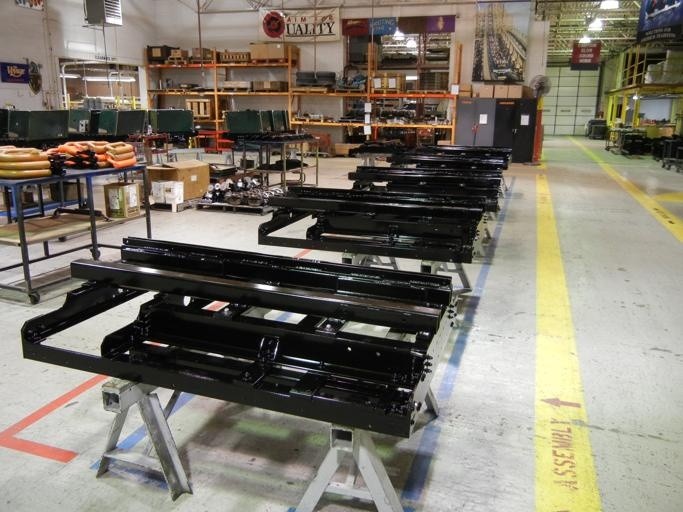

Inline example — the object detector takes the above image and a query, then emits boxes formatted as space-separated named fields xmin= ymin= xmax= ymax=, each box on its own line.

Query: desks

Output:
xmin=0 ymin=164 xmax=152 ymax=305
xmin=226 ymin=137 xmax=321 ymax=192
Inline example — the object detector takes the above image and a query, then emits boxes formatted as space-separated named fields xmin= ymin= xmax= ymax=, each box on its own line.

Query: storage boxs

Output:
xmin=104 ymin=183 xmax=140 ymax=218
xmin=22 ymin=192 xmax=34 ymax=204
xmin=460 ymin=84 xmax=471 ymax=98
xmin=479 ymin=85 xmax=493 ymax=98
xmin=493 ymin=85 xmax=509 ymax=98
xmin=145 ymin=160 xmax=210 ymax=204
xmin=0 ymin=109 xmax=194 ymax=141
xmin=508 ymin=85 xmax=533 ymax=98
xmin=49 ymin=181 xmax=84 ymax=201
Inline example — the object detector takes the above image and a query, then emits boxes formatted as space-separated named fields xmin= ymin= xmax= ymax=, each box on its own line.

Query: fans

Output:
xmin=529 ymin=75 xmax=552 ymax=98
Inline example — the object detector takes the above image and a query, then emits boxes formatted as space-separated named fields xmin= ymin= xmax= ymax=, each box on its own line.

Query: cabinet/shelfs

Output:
xmin=454 ymin=95 xmax=496 ymax=146
xmin=493 ymin=98 xmax=537 ymax=163
